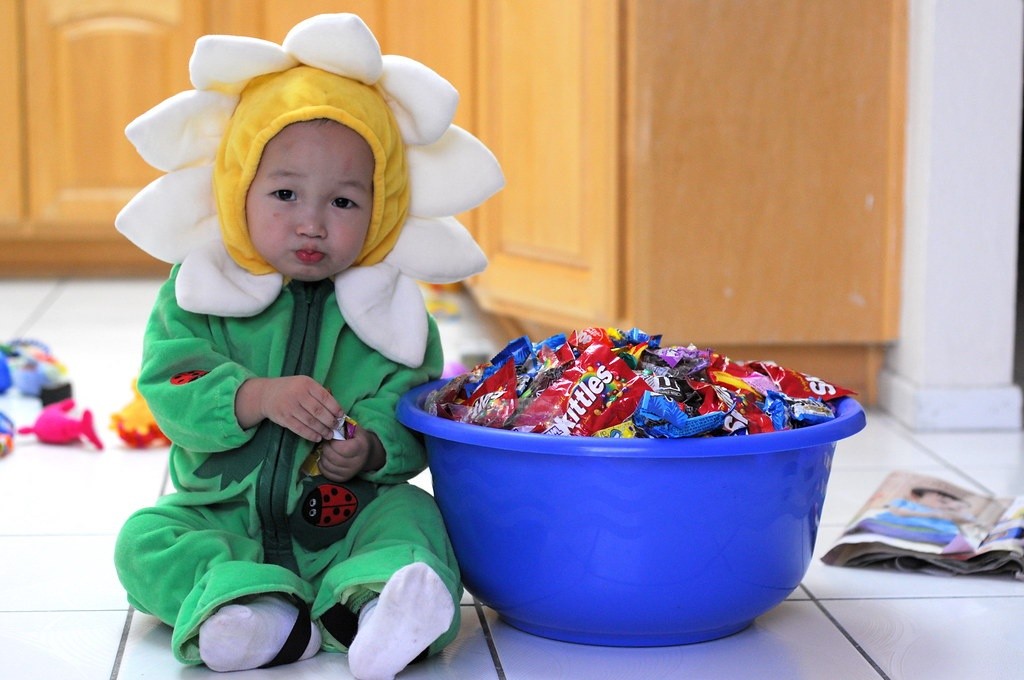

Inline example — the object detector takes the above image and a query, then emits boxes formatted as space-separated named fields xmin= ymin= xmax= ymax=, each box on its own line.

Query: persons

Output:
xmin=856 ymin=488 xmax=974 ymax=545
xmin=114 ymin=13 xmax=506 ymax=680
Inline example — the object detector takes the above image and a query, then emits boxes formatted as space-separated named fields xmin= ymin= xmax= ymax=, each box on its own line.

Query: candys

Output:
xmin=328 ymin=414 xmax=358 ymax=440
xmin=425 ymin=325 xmax=860 ymax=439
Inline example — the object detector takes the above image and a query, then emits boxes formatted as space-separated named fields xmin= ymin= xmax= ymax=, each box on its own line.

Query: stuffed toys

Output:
xmin=110 ymin=379 xmax=173 ymax=447
xmin=0 ymin=413 xmax=16 ymax=457
xmin=0 ymin=337 xmax=74 ymax=405
xmin=19 ymin=399 xmax=104 ymax=448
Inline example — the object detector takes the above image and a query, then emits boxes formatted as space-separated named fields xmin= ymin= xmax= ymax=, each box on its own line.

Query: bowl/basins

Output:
xmin=394 ymin=376 xmax=870 ymax=647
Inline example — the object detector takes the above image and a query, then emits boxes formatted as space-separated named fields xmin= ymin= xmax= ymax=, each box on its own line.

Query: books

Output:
xmin=821 ymin=472 xmax=1024 ymax=581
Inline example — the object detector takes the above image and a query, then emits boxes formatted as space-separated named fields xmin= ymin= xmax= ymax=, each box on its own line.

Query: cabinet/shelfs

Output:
xmin=0 ymin=0 xmax=905 ymax=408
